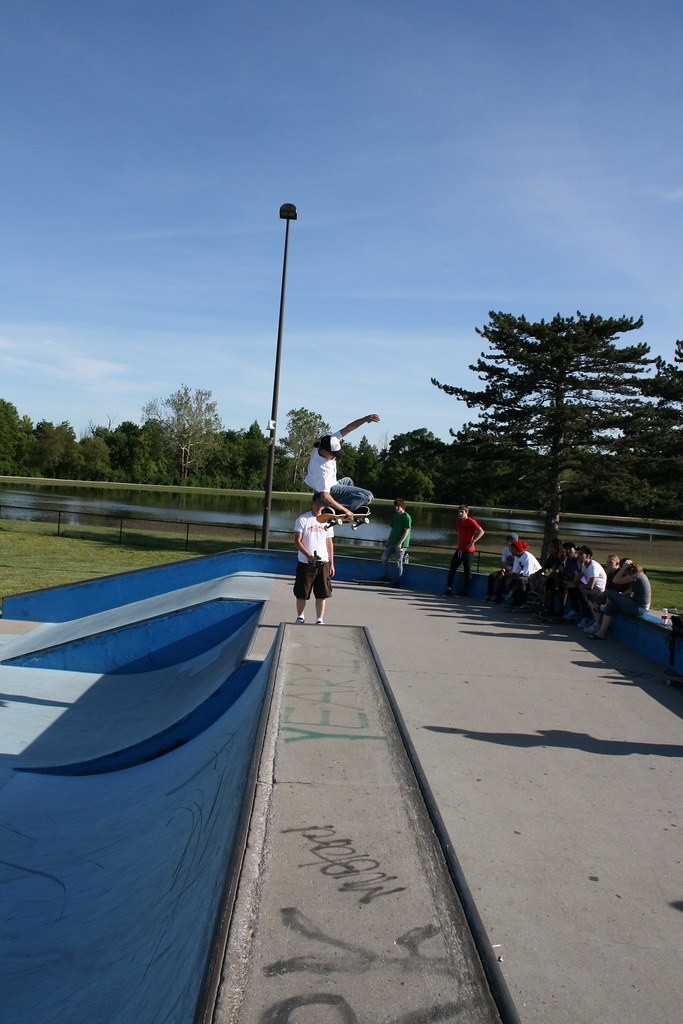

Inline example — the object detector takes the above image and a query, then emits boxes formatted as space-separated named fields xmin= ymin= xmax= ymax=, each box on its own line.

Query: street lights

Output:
xmin=261 ymin=202 xmax=297 ymax=549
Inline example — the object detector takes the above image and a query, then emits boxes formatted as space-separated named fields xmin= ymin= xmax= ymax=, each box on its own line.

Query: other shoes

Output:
xmin=481 ymin=594 xmax=499 ymax=604
xmin=321 ymin=505 xmax=336 ymax=514
xmin=577 ymin=617 xmax=585 ymax=627
xmin=584 ymin=622 xmax=599 ymax=634
xmin=376 ymin=576 xmax=391 ymax=583
xmin=563 ymin=612 xmax=581 ymax=619
xmin=454 ymin=591 xmax=467 ymax=598
xmin=316 ymin=618 xmax=324 ymax=624
xmin=442 ymin=590 xmax=451 ymax=597
xmin=295 ymin=616 xmax=305 ymax=623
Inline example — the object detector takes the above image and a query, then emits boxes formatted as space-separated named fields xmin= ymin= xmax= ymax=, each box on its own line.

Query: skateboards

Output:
xmin=353 ymin=578 xmax=390 ymax=585
xmin=664 ymin=669 xmax=683 ymax=686
xmin=527 ymin=591 xmax=546 ymax=618
xmin=317 ymin=513 xmax=370 ymax=531
xmin=545 ymin=616 xmax=579 ymax=624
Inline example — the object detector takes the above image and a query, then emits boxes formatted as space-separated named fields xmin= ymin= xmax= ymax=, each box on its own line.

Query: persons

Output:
xmin=294 ymin=494 xmax=334 ymax=624
xmin=304 ymin=414 xmax=381 ymax=521
xmin=521 ymin=539 xmax=607 ymax=628
xmin=442 ymin=505 xmax=484 ymax=598
xmin=377 ymin=498 xmax=411 ymax=588
xmin=584 ymin=554 xmax=651 ymax=640
xmin=482 ymin=533 xmax=541 ymax=607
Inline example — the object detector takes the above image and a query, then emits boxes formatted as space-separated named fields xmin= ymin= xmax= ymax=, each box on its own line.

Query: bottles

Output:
xmin=405 ymin=554 xmax=410 ymax=564
xmin=661 ymin=608 xmax=668 ymax=625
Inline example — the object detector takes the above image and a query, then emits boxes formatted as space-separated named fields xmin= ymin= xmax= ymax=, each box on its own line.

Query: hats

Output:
xmin=507 ymin=532 xmax=518 ymax=542
xmin=514 ymin=541 xmax=529 ymax=554
xmin=577 ymin=545 xmax=592 ymax=556
xmin=563 ymin=542 xmax=579 ymax=551
xmin=320 ymin=435 xmax=346 ymax=458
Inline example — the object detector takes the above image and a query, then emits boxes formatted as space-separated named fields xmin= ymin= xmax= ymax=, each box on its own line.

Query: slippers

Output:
xmin=593 ymin=605 xmax=605 ymax=614
xmin=584 ymin=633 xmax=605 ymax=640
